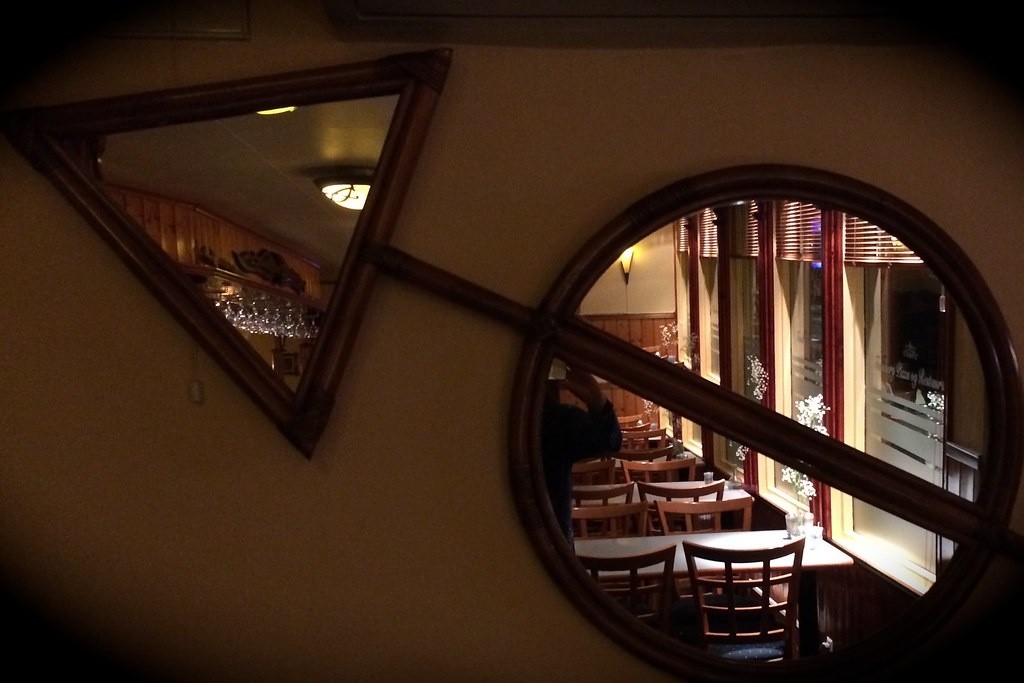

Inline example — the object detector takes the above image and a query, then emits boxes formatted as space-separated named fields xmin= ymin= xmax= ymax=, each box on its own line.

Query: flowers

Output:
xmin=781 ymin=393 xmax=831 ymax=513
xmin=642 ymin=398 xmax=657 ymax=418
xmin=659 ymin=321 xmax=701 ymax=435
xmin=729 ymin=355 xmax=770 ymax=461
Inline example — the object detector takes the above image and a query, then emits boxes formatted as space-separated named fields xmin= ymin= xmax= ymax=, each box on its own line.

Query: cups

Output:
xmin=703 ymin=472 xmax=713 ymax=484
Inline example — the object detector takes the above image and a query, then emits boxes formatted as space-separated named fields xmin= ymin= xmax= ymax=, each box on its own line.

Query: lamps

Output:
xmin=620 ymin=250 xmax=633 ymax=285
xmin=310 ymin=169 xmax=372 ymax=209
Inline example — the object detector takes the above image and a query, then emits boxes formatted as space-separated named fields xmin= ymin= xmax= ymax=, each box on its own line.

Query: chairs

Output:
xmin=577 ymin=543 xmax=677 ymax=635
xmin=572 ymin=499 xmax=649 ymax=538
xmin=682 ymin=537 xmax=805 ymax=665
xmin=619 ymin=428 xmax=666 ymax=451
xmin=572 ymin=481 xmax=635 ymax=507
xmin=621 ymin=457 xmax=697 ymax=482
xmin=569 ymin=479 xmax=752 ymax=505
xmin=654 ymin=496 xmax=755 ymax=598
xmin=572 ymin=459 xmax=616 ymax=484
xmin=637 ymin=480 xmax=724 ymax=531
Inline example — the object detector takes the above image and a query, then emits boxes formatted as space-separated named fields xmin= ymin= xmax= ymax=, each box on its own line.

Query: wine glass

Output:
xmin=215 ymin=295 xmax=319 ymax=338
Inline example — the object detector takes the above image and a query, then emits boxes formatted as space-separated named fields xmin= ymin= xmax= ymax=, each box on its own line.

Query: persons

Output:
xmin=542 ymin=362 xmax=622 ymax=562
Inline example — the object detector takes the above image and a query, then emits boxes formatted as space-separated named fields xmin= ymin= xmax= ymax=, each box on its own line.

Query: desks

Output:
xmin=574 ymin=529 xmax=854 ymax=583
xmin=591 ymin=451 xmax=706 ymax=469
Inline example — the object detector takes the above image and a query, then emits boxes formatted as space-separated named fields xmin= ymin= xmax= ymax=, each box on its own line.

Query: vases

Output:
xmin=728 ymin=464 xmax=744 ymax=490
xmin=786 ymin=512 xmax=814 ymax=541
xmin=671 ymin=440 xmax=684 ymax=458
